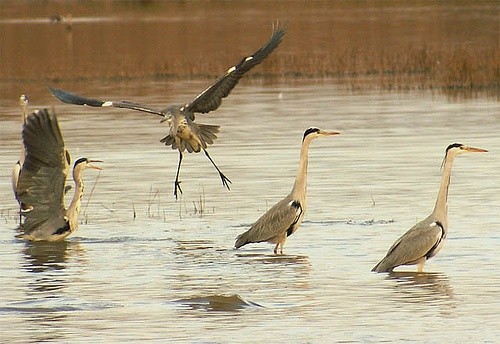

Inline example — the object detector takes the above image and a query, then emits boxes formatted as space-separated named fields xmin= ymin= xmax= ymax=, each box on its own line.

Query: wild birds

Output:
xmin=370 ymin=143 xmax=490 ymax=276
xmin=12 ymin=107 xmax=105 ymax=243
xmin=233 ymin=127 xmax=342 ymax=254
xmin=49 ymin=19 xmax=287 ymax=201
